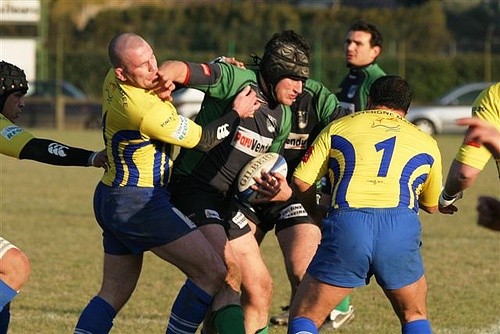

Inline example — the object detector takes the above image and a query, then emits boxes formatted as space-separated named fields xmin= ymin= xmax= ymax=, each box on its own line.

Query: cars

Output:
xmin=16 ymin=81 xmax=103 ymax=130
xmin=406 ymin=83 xmax=492 ymax=137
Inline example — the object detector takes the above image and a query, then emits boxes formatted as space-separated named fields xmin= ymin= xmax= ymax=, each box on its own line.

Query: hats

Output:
xmin=0 ymin=59 xmax=30 ymax=113
xmin=259 ymin=29 xmax=310 ymax=103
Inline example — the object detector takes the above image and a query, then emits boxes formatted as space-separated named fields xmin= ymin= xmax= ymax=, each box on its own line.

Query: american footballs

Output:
xmin=236 ymin=152 xmax=288 ymax=203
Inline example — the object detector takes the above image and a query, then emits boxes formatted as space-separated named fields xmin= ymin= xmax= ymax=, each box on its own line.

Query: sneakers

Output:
xmin=270 ymin=304 xmax=290 ymax=327
xmin=324 ymin=304 xmax=356 ymax=330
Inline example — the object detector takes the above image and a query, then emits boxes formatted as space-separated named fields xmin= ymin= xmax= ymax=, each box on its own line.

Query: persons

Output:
xmin=0 ymin=232 xmax=32 ymax=334
xmin=286 ymin=73 xmax=445 ymax=333
xmin=71 ymin=31 xmax=260 ymax=334
xmin=438 ymin=80 xmax=500 ymax=215
xmin=270 ymin=20 xmax=389 ymax=327
xmin=151 ymin=28 xmax=357 ymax=334
xmin=0 ymin=61 xmax=111 ymax=173
